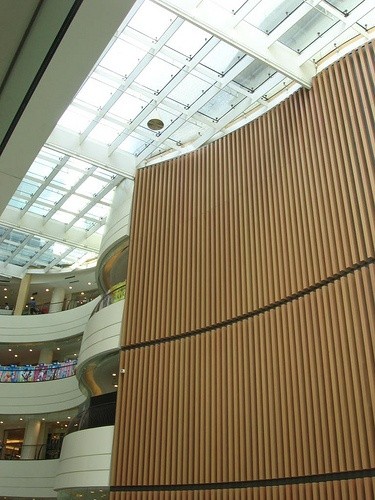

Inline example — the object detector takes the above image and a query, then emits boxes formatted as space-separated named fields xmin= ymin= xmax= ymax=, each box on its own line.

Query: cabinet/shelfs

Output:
xmin=0 ymin=309 xmax=13 ymax=315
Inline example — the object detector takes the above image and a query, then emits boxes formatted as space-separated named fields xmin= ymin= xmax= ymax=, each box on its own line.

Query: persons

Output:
xmin=0 ymin=295 xmax=93 ymax=316
